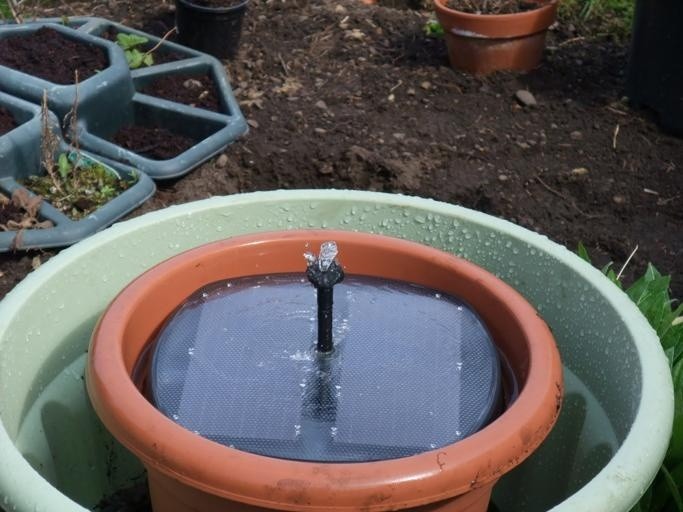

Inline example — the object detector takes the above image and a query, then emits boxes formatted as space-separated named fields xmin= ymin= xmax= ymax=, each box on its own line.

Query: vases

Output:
xmin=434 ymin=1 xmax=559 ymax=74
xmin=85 ymin=227 xmax=565 ymax=510
xmin=175 ymin=0 xmax=249 ymax=61
